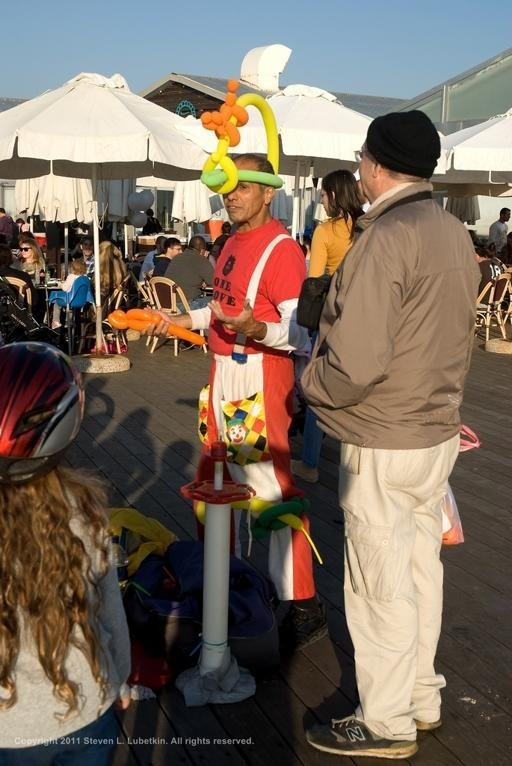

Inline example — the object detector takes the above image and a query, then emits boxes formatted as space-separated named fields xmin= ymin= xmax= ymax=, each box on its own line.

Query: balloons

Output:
xmin=198 ymin=78 xmax=283 ymax=195
xmin=194 ymin=495 xmax=325 ymax=567
xmin=127 ymin=190 xmax=154 ymax=228
xmin=107 ymin=308 xmax=208 ymax=347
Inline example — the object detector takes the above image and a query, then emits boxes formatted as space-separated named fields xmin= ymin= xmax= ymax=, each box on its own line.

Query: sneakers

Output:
xmin=276 ymin=599 xmax=330 ymax=658
xmin=413 ymin=710 xmax=443 ymax=731
xmin=305 ymin=708 xmax=419 ymax=761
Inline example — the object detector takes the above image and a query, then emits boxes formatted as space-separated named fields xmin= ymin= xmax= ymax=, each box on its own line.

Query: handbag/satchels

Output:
xmin=91 ymin=339 xmax=128 ymax=353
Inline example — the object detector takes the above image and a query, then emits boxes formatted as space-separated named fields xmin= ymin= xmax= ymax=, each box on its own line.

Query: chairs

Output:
xmin=1 ymin=211 xmax=512 ymax=381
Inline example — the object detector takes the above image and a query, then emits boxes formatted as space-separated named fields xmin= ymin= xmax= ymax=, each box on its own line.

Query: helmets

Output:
xmin=0 ymin=340 xmax=86 ymax=488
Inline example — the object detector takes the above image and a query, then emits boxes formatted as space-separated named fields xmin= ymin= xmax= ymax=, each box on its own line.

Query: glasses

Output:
xmin=20 ymin=247 xmax=33 ymax=252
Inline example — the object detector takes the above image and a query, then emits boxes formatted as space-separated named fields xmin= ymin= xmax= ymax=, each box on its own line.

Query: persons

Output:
xmin=474 ymin=245 xmax=502 ymax=311
xmin=1 ymin=339 xmax=132 ymax=766
xmin=487 ymin=207 xmax=510 ymax=256
xmin=295 ymin=109 xmax=483 ymax=762
xmin=289 ymin=169 xmax=366 ymax=484
xmin=1 ymin=206 xmax=240 ymax=358
xmin=142 ymin=152 xmax=328 ymax=655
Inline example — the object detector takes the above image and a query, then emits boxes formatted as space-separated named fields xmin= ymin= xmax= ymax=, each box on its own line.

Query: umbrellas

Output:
xmin=0 ymin=69 xmax=236 ymax=371
xmin=5 ymin=174 xmax=107 ymax=286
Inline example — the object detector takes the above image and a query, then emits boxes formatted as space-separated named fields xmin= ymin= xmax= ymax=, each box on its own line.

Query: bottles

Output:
xmin=35 ymin=266 xmax=50 ymax=286
